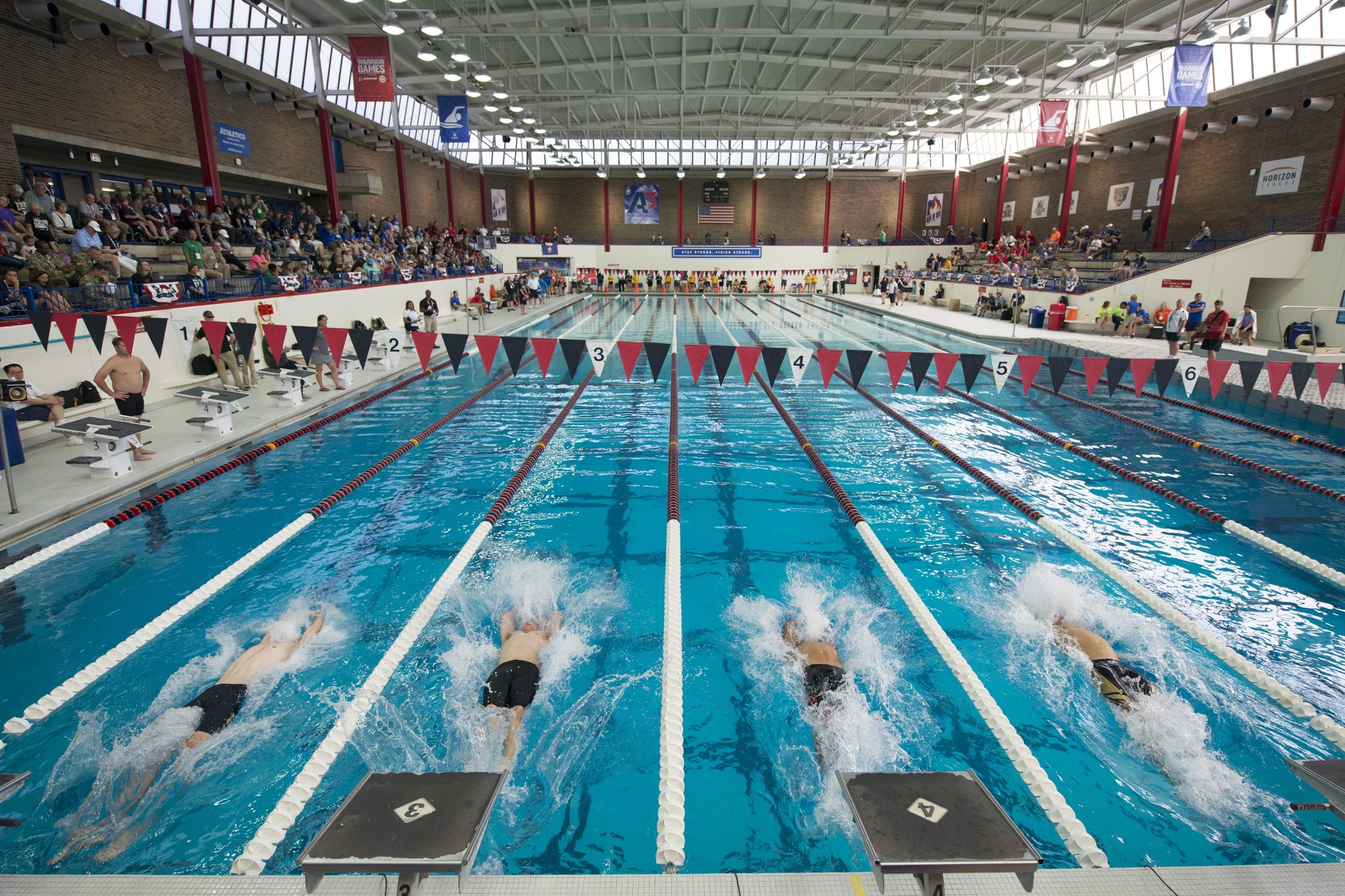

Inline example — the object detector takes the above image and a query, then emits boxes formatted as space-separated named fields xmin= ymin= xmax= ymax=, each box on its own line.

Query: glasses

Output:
xmin=206 ymin=316 xmax=214 ymax=318
xmin=7 ymin=371 xmax=24 ymax=375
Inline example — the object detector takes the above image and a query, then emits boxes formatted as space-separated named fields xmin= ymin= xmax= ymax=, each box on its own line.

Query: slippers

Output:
xmin=319 ymin=387 xmax=346 ymax=391
xmin=247 ymin=268 xmax=257 ymax=275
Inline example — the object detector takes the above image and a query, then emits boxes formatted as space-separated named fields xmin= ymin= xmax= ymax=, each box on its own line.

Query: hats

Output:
xmin=1010 ymin=242 xmax=1015 ymax=246
xmin=371 ymin=214 xmax=376 ymax=217
xmin=1070 ymin=227 xmax=1075 ymax=229
xmin=554 ymin=227 xmax=557 ymax=229
xmin=422 ymin=250 xmax=427 ymax=254
xmin=407 ymin=226 xmax=412 ymax=231
xmin=218 ymin=229 xmax=229 ymax=238
xmin=21 ymin=166 xmax=33 ymax=171
xmin=1106 ymin=224 xmax=1113 ymax=227
xmin=986 ymin=252 xmax=991 ymax=256
xmin=381 ymin=217 xmax=384 ymax=219
xmin=408 ymin=260 xmax=413 ymax=264
xmin=89 ymin=220 xmax=102 ymax=233
xmin=1144 ymin=209 xmax=1152 ymax=213
xmin=301 ymin=261 xmax=307 ymax=264
xmin=1082 ymin=225 xmax=1089 ymax=229
xmin=367 ymin=259 xmax=373 ymax=264
xmin=484 ymin=304 xmax=488 ymax=308
xmin=345 ymin=248 xmax=350 ymax=251
xmin=566 ymin=236 xmax=568 ymax=237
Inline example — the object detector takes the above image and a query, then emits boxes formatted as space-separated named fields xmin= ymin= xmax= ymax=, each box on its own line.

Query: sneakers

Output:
xmin=56 ymin=419 xmax=83 ymax=445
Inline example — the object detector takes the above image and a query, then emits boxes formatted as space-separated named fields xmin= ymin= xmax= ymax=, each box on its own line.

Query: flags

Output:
xmin=698 ymin=205 xmax=734 ymax=225
xmin=437 ymin=94 xmax=470 ymax=143
xmin=1166 ymin=45 xmax=1213 ymax=107
xmin=348 ymin=35 xmax=394 ymax=102
xmin=1035 ymin=100 xmax=1069 ymax=147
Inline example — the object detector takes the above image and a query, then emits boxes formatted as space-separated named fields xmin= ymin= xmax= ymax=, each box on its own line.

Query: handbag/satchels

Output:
xmin=1056 ymin=255 xmax=1062 ymax=259
xmin=54 ymin=388 xmax=84 ymax=409
xmin=63 ymin=226 xmax=76 ymax=235
xmin=1007 ymin=307 xmax=1013 ymax=320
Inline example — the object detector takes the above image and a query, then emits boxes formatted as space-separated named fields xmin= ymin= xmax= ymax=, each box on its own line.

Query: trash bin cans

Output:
xmin=1288 ymin=321 xmax=1319 ymax=349
xmin=1028 ymin=305 xmax=1047 ymax=328
xmin=1047 ymin=303 xmax=1066 ymax=330
xmin=1064 ymin=306 xmax=1078 ymax=321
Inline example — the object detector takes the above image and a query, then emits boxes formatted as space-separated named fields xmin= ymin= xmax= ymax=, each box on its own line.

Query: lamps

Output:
xmin=946 ymin=40 xmax=991 ymax=102
xmin=379 ymin=5 xmax=808 ymax=182
xmin=849 ymin=145 xmax=875 ymax=158
xmin=903 ymin=98 xmax=942 ymax=128
xmin=872 ymin=130 xmax=904 ymax=144
xmin=923 ymin=99 xmax=963 ymax=116
xmin=1056 ymin=41 xmax=1110 ymax=68
xmin=839 ymin=152 xmax=865 ymax=164
xmin=975 ymin=40 xmax=1023 ymax=85
xmin=1194 ymin=0 xmax=1255 ymax=48
xmin=831 ymin=153 xmax=854 ymax=169
xmin=859 ymin=132 xmax=889 ymax=153
xmin=887 ymin=98 xmax=921 ymax=137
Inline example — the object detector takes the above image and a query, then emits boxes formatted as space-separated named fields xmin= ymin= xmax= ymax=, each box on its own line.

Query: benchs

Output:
xmin=435 ymin=312 xmax=456 ymax=325
xmin=18 ymin=395 xmax=115 ymax=440
xmin=163 ymin=362 xmax=261 ymax=391
xmin=457 ymin=305 xmax=479 ymax=320
xmin=348 ymin=327 xmax=390 ymax=343
xmin=488 ymin=299 xmax=507 ymax=310
xmin=891 ymin=248 xmax=1238 ymax=342
xmin=9 ymin=230 xmax=365 ymax=307
xmin=286 ymin=348 xmax=303 ymax=358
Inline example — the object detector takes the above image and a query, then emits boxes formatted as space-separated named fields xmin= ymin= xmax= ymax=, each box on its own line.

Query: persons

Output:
xmin=1052 ymin=614 xmax=1159 ymax=713
xmin=479 ymin=610 xmax=562 ymax=770
xmin=0 ymin=164 xmax=1254 ymax=461
xmin=46 ymin=609 xmax=323 ymax=864
xmin=783 ymin=620 xmax=846 ymax=775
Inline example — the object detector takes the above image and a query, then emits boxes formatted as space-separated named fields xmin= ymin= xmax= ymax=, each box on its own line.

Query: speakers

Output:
xmin=928 ymin=140 xmax=935 ymax=145
xmin=502 ymin=136 xmax=511 ymax=143
xmin=416 ymin=95 xmax=425 ymax=104
xmin=1265 ymin=3 xmax=1288 ymax=19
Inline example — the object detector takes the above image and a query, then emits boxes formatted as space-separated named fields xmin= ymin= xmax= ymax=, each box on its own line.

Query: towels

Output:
xmin=1230 ymin=308 xmax=1258 ymax=341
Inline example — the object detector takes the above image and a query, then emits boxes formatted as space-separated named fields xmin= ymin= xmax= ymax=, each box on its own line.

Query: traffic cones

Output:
xmin=744 ymin=281 xmax=749 ymax=293
xmin=716 ymin=281 xmax=720 ymax=293
xmin=662 ymin=284 xmax=666 ymax=293
xmin=770 ymin=282 xmax=775 ymax=293
xmin=685 ymin=284 xmax=689 ymax=293
xmin=604 ymin=281 xmax=609 ymax=293
xmin=798 ymin=284 xmax=802 ymax=293
xmin=635 ymin=283 xmax=639 ymax=293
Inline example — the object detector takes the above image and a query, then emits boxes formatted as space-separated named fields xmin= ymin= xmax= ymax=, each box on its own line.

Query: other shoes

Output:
xmin=976 ymin=270 xmax=990 ymax=274
xmin=546 ymin=292 xmax=577 ymax=298
xmin=1081 ymin=257 xmax=1087 ymax=260
xmin=689 ymin=291 xmax=694 ymax=293
xmin=497 ymin=300 xmax=545 ymax=312
xmin=1009 ymin=321 xmax=1019 ymax=324
xmin=673 ymin=291 xmax=679 ymax=293
xmin=1184 ymin=247 xmax=1191 ymax=250
xmin=839 ymin=294 xmax=845 ymax=296
xmin=223 ymin=283 xmax=236 ymax=288
xmin=302 ymin=394 xmax=312 ymax=400
xmin=952 ymin=262 xmax=956 ymax=264
xmin=433 ymin=344 xmax=440 ymax=348
xmin=780 ymin=291 xmax=786 ymax=293
xmin=1092 ymin=328 xmax=1135 ymax=339
xmin=725 ymin=291 xmax=732 ymax=293
xmin=1087 ymin=256 xmax=1093 ymax=260
xmin=151 ymin=271 xmax=161 ymax=280
xmin=972 ymin=313 xmax=996 ymax=319
xmin=224 ymin=374 xmax=272 ymax=392
xmin=117 ymin=277 xmax=122 ymax=281
xmin=161 ymin=239 xmax=175 ymax=245
xmin=472 ymin=311 xmax=494 ymax=320
xmin=881 ymin=302 xmax=898 ymax=309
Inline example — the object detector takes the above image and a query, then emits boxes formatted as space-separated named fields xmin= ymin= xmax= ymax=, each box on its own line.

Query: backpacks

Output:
xmin=375 ymin=317 xmax=387 ymax=330
xmin=191 ymin=354 xmax=214 ymax=375
xmin=1000 ymin=310 xmax=1007 ymax=320
xmin=80 ymin=380 xmax=101 ymax=403
xmin=355 ymin=320 xmax=367 ymax=330
xmin=1144 ymin=313 xmax=1149 ymax=324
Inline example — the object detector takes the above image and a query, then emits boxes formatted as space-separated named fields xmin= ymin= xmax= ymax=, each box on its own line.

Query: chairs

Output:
xmin=790 ymin=283 xmax=803 ymax=292
xmin=608 ymin=282 xmax=644 ymax=292
xmin=658 ymin=282 xmax=669 ymax=292
